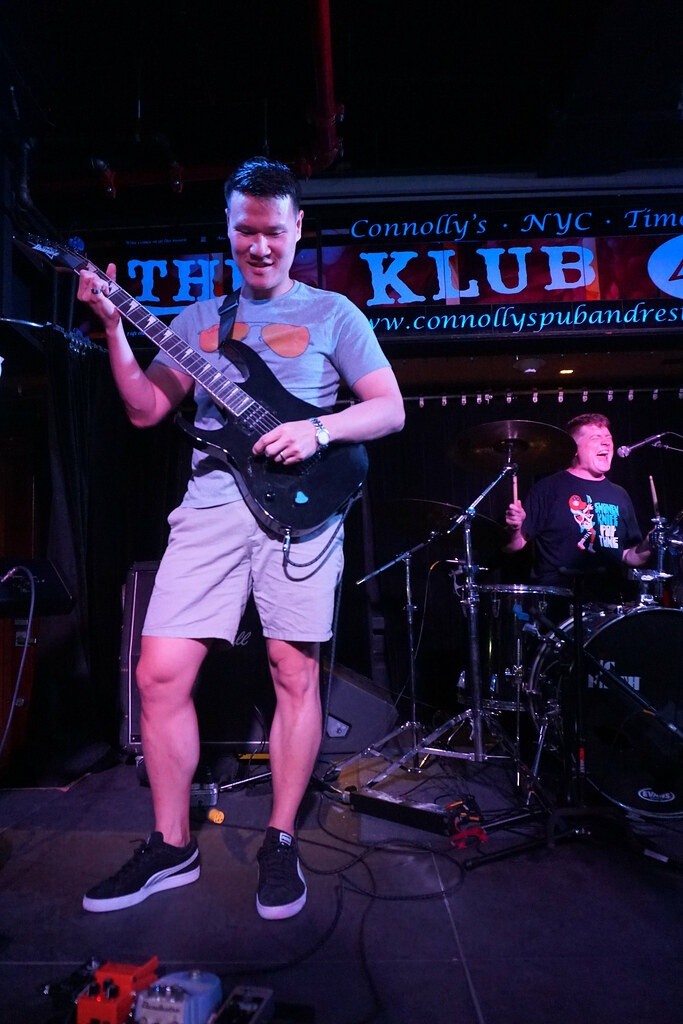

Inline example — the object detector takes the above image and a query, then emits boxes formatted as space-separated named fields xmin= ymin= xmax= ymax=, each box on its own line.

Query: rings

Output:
xmin=507 ymin=510 xmax=510 ymax=514
xmin=91 ymin=288 xmax=102 ymax=294
xmin=280 ymin=451 xmax=286 ymax=462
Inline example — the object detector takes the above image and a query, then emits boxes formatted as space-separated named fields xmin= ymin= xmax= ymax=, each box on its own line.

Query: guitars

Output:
xmin=10 ymin=226 xmax=373 ymax=545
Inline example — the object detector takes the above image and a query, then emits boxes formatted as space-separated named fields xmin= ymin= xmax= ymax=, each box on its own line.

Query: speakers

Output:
xmin=117 ymin=557 xmax=278 ymax=753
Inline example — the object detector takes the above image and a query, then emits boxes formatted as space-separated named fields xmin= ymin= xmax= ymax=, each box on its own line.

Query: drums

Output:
xmin=448 ymin=582 xmax=575 ymax=714
xmin=525 ymin=601 xmax=683 ymax=821
xmin=659 ymin=511 xmax=683 ymax=607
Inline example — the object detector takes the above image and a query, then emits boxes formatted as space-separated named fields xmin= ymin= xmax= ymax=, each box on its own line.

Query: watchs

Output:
xmin=307 ymin=417 xmax=330 ymax=453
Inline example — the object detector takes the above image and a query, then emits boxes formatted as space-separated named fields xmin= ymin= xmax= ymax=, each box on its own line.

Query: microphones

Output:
xmin=617 ymin=434 xmax=666 ymax=458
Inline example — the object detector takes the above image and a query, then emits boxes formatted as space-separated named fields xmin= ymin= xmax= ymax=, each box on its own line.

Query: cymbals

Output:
xmin=372 ymin=496 xmax=506 ymax=547
xmin=441 ymin=419 xmax=578 ymax=477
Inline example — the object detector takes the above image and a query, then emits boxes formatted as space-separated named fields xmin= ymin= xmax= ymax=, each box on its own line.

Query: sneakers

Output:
xmin=255 ymin=826 xmax=307 ymax=920
xmin=83 ymin=831 xmax=199 ymax=911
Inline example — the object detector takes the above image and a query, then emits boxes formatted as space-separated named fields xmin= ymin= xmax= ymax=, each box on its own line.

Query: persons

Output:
xmin=505 ymin=412 xmax=655 ymax=608
xmin=76 ymin=160 xmax=407 ymax=921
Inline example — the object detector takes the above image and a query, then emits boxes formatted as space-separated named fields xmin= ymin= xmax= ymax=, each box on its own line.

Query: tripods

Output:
xmin=325 ymin=528 xmax=487 ymax=785
xmin=363 ymin=518 xmax=558 ymax=815
